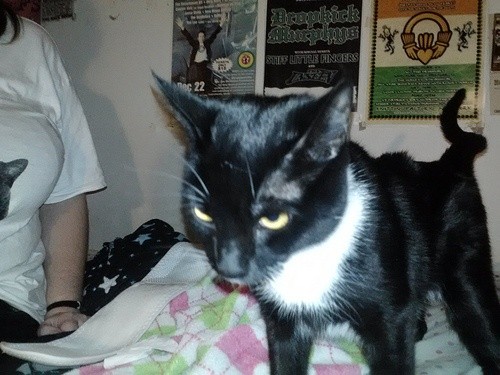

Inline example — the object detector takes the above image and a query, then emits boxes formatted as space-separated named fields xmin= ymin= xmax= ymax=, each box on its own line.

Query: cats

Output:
xmin=121 ymin=68 xmax=500 ymax=375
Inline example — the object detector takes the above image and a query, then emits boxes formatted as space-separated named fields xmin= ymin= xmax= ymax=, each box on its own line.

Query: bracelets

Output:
xmin=47 ymin=300 xmax=82 ymax=311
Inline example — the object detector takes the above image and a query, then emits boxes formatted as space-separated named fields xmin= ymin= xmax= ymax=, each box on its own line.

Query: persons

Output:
xmin=175 ymin=11 xmax=227 ymax=96
xmin=0 ymin=0 xmax=106 ymax=375
